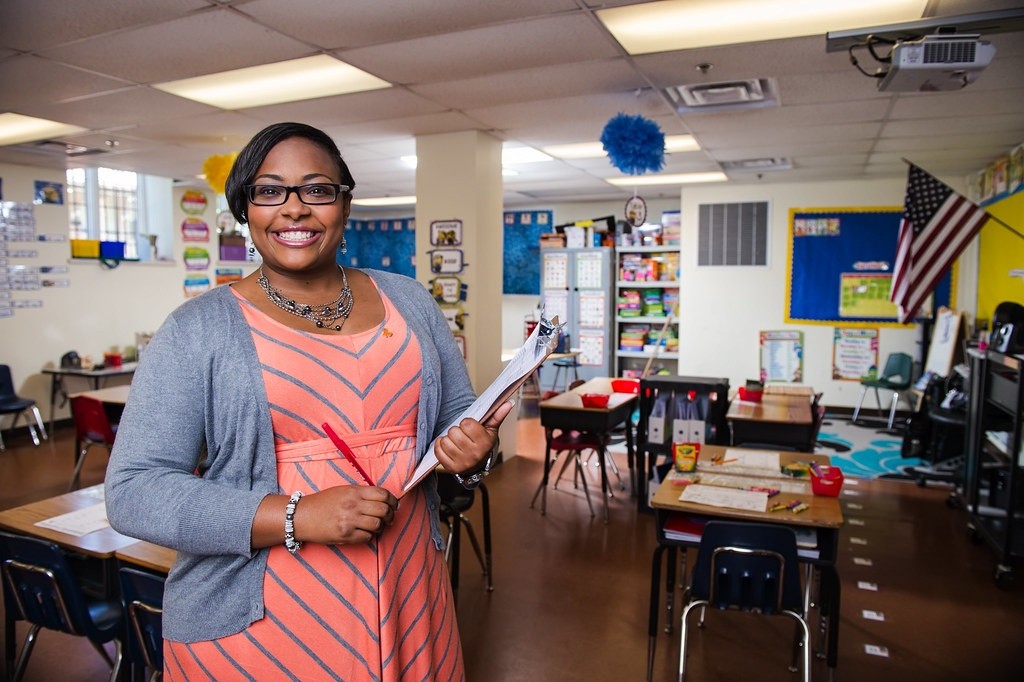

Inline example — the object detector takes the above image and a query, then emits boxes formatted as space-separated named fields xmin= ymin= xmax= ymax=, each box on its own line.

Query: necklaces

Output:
xmin=256 ymin=263 xmax=353 ymax=331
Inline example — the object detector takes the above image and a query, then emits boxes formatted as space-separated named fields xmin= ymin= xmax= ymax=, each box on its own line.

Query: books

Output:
xmin=621 ymin=253 xmax=680 ymax=281
xmin=621 ymin=323 xmax=678 ymax=351
xmin=617 ymin=288 xmax=678 ymax=317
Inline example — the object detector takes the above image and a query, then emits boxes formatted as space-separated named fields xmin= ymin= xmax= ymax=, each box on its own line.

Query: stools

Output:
xmin=552 ymin=360 xmax=582 ymax=389
xmin=516 ymin=365 xmax=543 ymax=421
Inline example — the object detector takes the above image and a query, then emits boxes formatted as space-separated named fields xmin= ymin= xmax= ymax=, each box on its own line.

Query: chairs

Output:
xmin=0 ymin=531 xmax=169 ymax=682
xmin=435 ymin=435 xmax=494 ymax=599
xmin=811 ymin=391 xmax=826 ymax=450
xmin=530 ymin=381 xmax=625 ymax=520
xmin=677 ymin=520 xmax=812 ymax=682
xmin=68 ymin=394 xmax=119 ymax=493
xmin=852 ymin=353 xmax=914 ymax=432
xmin=0 ymin=365 xmax=47 ymax=448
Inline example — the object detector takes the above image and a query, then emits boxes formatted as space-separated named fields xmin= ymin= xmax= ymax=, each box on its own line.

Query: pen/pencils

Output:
xmin=322 ymin=423 xmax=377 ymax=486
xmin=711 ymin=458 xmax=737 ymax=466
xmin=809 ymin=460 xmax=826 ymax=477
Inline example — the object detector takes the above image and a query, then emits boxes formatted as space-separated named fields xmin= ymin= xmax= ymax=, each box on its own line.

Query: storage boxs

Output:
xmin=71 ymin=239 xmax=125 ymax=259
xmin=649 ymin=414 xmax=709 ymax=511
xmin=539 ymin=233 xmax=566 ymax=247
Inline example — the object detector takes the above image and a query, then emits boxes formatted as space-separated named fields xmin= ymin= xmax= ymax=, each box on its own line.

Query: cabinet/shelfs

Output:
xmin=540 ymin=246 xmax=614 ymax=393
xmin=638 ymin=375 xmax=728 ymax=514
xmin=961 ymin=348 xmax=1024 ymax=592
xmin=615 ymin=246 xmax=681 ymax=378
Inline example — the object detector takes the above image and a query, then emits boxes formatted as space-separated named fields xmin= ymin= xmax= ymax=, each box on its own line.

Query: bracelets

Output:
xmin=283 ymin=490 xmax=303 ymax=555
xmin=455 ymin=454 xmax=492 ymax=488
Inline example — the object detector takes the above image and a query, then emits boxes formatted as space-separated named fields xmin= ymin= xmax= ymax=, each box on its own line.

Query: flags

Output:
xmin=889 ymin=165 xmax=992 ymax=326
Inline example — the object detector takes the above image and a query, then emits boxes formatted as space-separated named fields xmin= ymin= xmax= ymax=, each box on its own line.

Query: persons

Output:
xmin=105 ymin=122 xmax=515 ymax=682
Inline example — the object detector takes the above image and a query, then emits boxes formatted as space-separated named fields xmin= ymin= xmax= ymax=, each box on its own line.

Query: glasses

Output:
xmin=241 ymin=184 xmax=349 ymax=205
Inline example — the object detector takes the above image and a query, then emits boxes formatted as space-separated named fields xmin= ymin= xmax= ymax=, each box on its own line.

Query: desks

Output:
xmin=0 ymin=480 xmax=179 ymax=604
xmin=724 ymin=383 xmax=816 ymax=452
xmin=43 ymin=361 xmax=141 ymax=485
xmin=515 ymin=345 xmax=583 ymax=382
xmin=540 ymin=376 xmax=640 ymax=526
xmin=646 ymin=445 xmax=843 ymax=682
